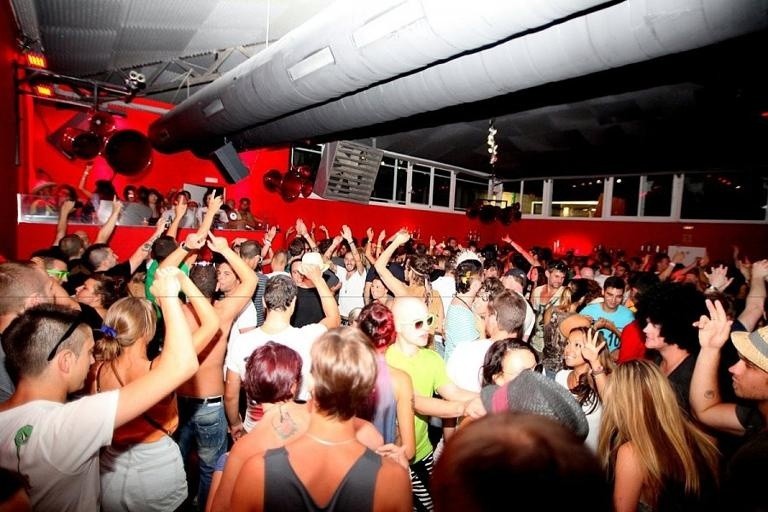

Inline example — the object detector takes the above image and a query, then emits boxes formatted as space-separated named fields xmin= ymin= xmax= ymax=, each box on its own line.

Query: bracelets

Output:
xmin=392 ymin=241 xmax=399 ymax=248
xmin=310 ymin=231 xmax=314 ymax=235
xmin=376 ymin=245 xmax=381 ymax=249
xmin=615 ymin=329 xmax=621 ymax=337
xmin=509 ymin=239 xmax=514 ymax=244
xmin=366 ymin=240 xmax=372 ymax=243
xmin=348 ymin=240 xmax=355 ymax=245
xmin=430 ymin=246 xmax=434 ymax=249
xmin=303 ymin=233 xmax=310 ymax=239
xmin=670 ymin=261 xmax=677 ymax=268
xmin=591 ymin=366 xmax=605 ymax=378
xmin=264 ymin=238 xmax=272 ymax=245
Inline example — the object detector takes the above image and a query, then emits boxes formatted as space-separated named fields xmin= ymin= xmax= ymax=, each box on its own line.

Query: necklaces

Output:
xmin=303 ymin=430 xmax=359 ymax=450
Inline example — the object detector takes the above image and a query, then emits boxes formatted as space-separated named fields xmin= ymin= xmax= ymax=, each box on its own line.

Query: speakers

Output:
xmin=214 ymin=141 xmax=249 ymax=184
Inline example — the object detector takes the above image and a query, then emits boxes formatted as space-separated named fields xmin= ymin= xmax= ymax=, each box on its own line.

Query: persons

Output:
xmin=721 ymin=259 xmax=767 ymax=399
xmin=360 ymin=278 xmax=394 ymax=335
xmin=0 ymin=274 xmax=199 ymax=512
xmin=684 ymin=271 xmax=705 ymax=291
xmin=524 ymin=266 xmax=544 ymax=288
xmin=530 ymin=259 xmax=573 ymax=366
xmin=354 ymin=303 xmax=414 ymax=464
xmin=205 ymin=450 xmax=231 ymax=512
xmin=413 ymin=235 xmax=437 ymax=257
xmin=580 ymin=265 xmax=602 ymax=304
xmin=1 ymin=465 xmax=32 ymax=512
xmin=229 ymin=325 xmax=412 ymax=511
xmin=211 ymin=340 xmax=384 ymax=512
xmin=500 ymin=266 xmax=534 ymax=342
xmin=629 ymin=253 xmax=642 ymax=275
xmin=81 ymin=215 xmax=168 ymax=293
xmin=283 ymin=219 xmax=317 ymax=272
xmin=57 ymin=194 xmax=124 ymax=296
xmin=578 ymin=276 xmax=635 ymax=353
xmin=264 ymin=249 xmax=290 ymax=286
xmin=0 ymin=263 xmax=56 ymax=404
xmin=216 ymin=259 xmax=257 ymax=339
xmin=293 ymin=251 xmax=330 ymax=328
xmin=166 ymin=193 xmax=188 ymax=241
xmin=614 ymin=251 xmax=626 ymax=263
xmin=502 ymin=250 xmax=526 ymax=285
xmin=444 ymin=259 xmax=486 ymax=359
xmin=445 ymin=237 xmax=457 ymax=254
xmin=592 ymin=248 xmax=610 ymax=274
xmin=426 ymin=410 xmax=610 ymax=512
xmin=459 ymin=338 xmax=538 ymax=431
xmin=222 ymin=261 xmax=341 ymax=443
xmin=636 ymin=281 xmax=698 ymax=411
xmin=40 ymin=257 xmax=69 ymax=287
xmin=625 ymin=285 xmax=641 ymax=313
xmin=30 ymin=255 xmax=123 ymax=344
xmin=32 ymin=200 xmax=91 ymax=264
xmin=466 ymin=242 xmax=480 ymax=257
xmin=376 ymin=230 xmax=385 ymax=260
xmin=90 ymin=265 xmax=221 ymax=512
xmin=319 ymin=224 xmax=332 ymax=250
xmin=501 ymin=231 xmax=550 ymax=280
xmin=650 ymin=252 xmax=685 ymax=281
xmin=552 ymin=326 xmax=613 ymax=453
xmin=481 ymin=244 xmax=499 ymax=279
xmin=596 ymin=358 xmax=721 ymax=512
xmin=238 ymin=239 xmax=270 ymax=330
xmin=380 ymin=298 xmax=488 ymax=512
xmin=675 ymin=257 xmax=709 ymax=280
xmin=29 ymin=160 xmax=269 ymax=230
xmin=367 ymin=227 xmax=374 ymax=269
xmin=373 ymin=229 xmax=446 ymax=350
xmin=144 ymin=189 xmax=224 ymax=354
xmin=611 ymin=262 xmax=629 ymax=293
xmin=324 ymin=225 xmax=366 ymax=325
xmin=686 ymin=298 xmax=768 ymax=512
xmin=162 ymin=231 xmax=258 ymax=500
xmin=704 ymin=243 xmax=762 ymax=298
xmin=231 ymin=225 xmax=277 ymax=267
xmin=544 ymin=279 xmax=606 ymax=376
xmin=447 ymin=289 xmax=528 ymax=392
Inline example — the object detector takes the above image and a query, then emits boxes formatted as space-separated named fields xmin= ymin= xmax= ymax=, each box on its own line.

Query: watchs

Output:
xmin=180 ymin=242 xmax=193 ymax=252
xmin=142 ymin=243 xmax=153 ymax=254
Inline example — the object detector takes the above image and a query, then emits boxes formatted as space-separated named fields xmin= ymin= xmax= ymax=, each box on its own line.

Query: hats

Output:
xmin=730 ymin=325 xmax=768 ymax=372
xmin=296 ymin=254 xmax=330 ymax=276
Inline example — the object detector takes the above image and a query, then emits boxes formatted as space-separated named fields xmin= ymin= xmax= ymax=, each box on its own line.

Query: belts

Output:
xmin=180 ymin=394 xmax=224 ymax=409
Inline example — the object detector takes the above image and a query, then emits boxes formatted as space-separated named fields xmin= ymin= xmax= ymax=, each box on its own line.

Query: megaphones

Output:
xmin=127 ymin=70 xmax=146 ymax=84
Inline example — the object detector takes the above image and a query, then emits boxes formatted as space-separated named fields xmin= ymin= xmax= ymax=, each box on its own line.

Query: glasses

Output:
xmin=43 ymin=269 xmax=70 ymax=282
xmin=47 ymin=312 xmax=89 ymax=362
xmin=399 ymin=315 xmax=434 ymax=330
xmin=553 ymin=264 xmax=571 ymax=272
